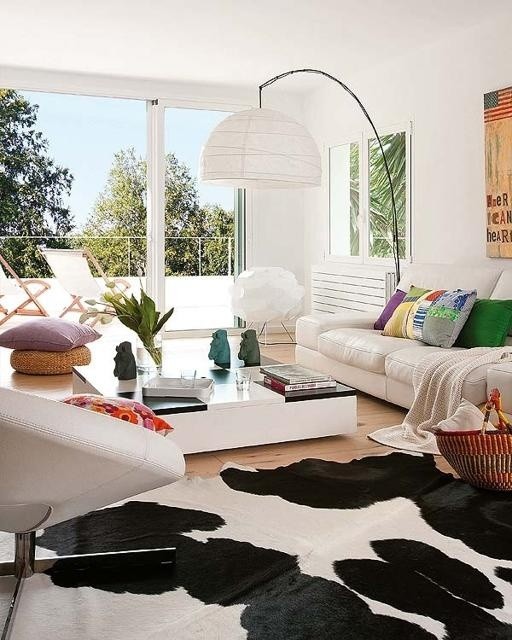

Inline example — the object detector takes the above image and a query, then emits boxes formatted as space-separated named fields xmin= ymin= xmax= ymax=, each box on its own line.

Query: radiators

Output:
xmin=310 ymin=265 xmax=397 ymax=319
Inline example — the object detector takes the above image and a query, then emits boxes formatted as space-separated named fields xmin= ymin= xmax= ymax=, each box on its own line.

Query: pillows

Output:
xmin=374 ymin=287 xmax=406 ymax=330
xmin=452 ymin=298 xmax=510 ymax=347
xmin=1 ymin=318 xmax=104 ymax=352
xmin=379 ymin=284 xmax=477 ymax=350
xmin=58 ymin=394 xmax=174 ymax=438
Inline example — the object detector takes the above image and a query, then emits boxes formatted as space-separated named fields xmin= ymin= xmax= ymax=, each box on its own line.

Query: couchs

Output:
xmin=294 ymin=262 xmax=511 ymax=414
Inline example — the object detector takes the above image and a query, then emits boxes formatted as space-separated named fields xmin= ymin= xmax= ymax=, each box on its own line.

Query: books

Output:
xmin=259 ymin=363 xmax=333 ymax=384
xmin=264 ymin=374 xmax=338 ymax=392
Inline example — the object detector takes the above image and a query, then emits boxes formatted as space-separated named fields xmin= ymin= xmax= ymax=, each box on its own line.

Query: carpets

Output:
xmin=0 ymin=447 xmax=511 ymax=639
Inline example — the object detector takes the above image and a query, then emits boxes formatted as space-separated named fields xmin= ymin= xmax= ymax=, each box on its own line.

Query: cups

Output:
xmin=180 ymin=370 xmax=197 ymax=388
xmin=236 ymin=370 xmax=252 ymax=395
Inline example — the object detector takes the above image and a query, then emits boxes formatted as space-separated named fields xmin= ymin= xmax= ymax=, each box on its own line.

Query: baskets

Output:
xmin=435 ymin=388 xmax=512 ymax=493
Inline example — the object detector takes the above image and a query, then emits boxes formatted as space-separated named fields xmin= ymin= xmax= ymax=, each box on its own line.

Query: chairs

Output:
xmin=0 ymin=389 xmax=185 ymax=637
xmin=0 ymin=252 xmax=50 ymax=324
xmin=36 ymin=244 xmax=132 ymax=318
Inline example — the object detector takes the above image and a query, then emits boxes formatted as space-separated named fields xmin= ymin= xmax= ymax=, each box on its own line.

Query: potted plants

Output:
xmin=86 ymin=268 xmax=176 ymax=373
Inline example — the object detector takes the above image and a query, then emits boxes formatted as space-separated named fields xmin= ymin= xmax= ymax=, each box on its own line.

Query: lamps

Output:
xmin=199 ymin=66 xmax=401 ymax=290
xmin=229 ymin=266 xmax=303 ymax=349
